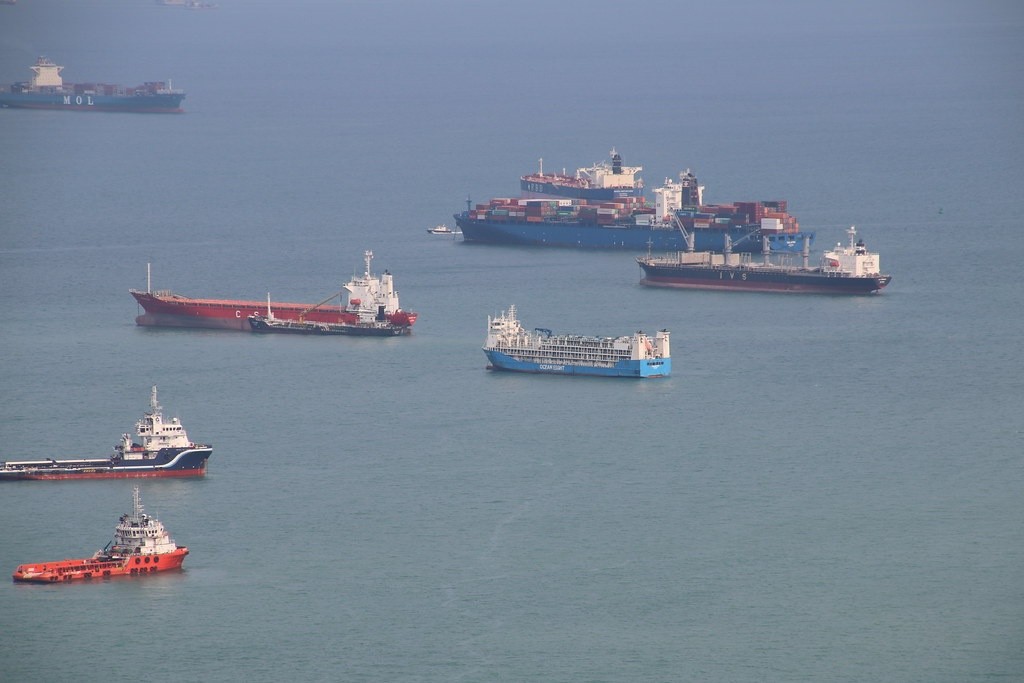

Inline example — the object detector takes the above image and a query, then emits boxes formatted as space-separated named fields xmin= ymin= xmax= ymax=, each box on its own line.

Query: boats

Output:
xmin=520 ymin=146 xmax=645 ymax=200
xmin=427 ymin=224 xmax=452 ymax=234
xmin=129 ymin=249 xmax=418 ymax=335
xmin=12 ymin=485 xmax=190 ymax=583
xmin=0 ymin=385 xmax=213 ymax=479
xmin=482 ymin=304 xmax=672 ymax=378
xmin=635 ymin=207 xmax=892 ymax=294
xmin=453 ymin=168 xmax=815 ymax=253
xmin=0 ymin=56 xmax=187 ymax=114
